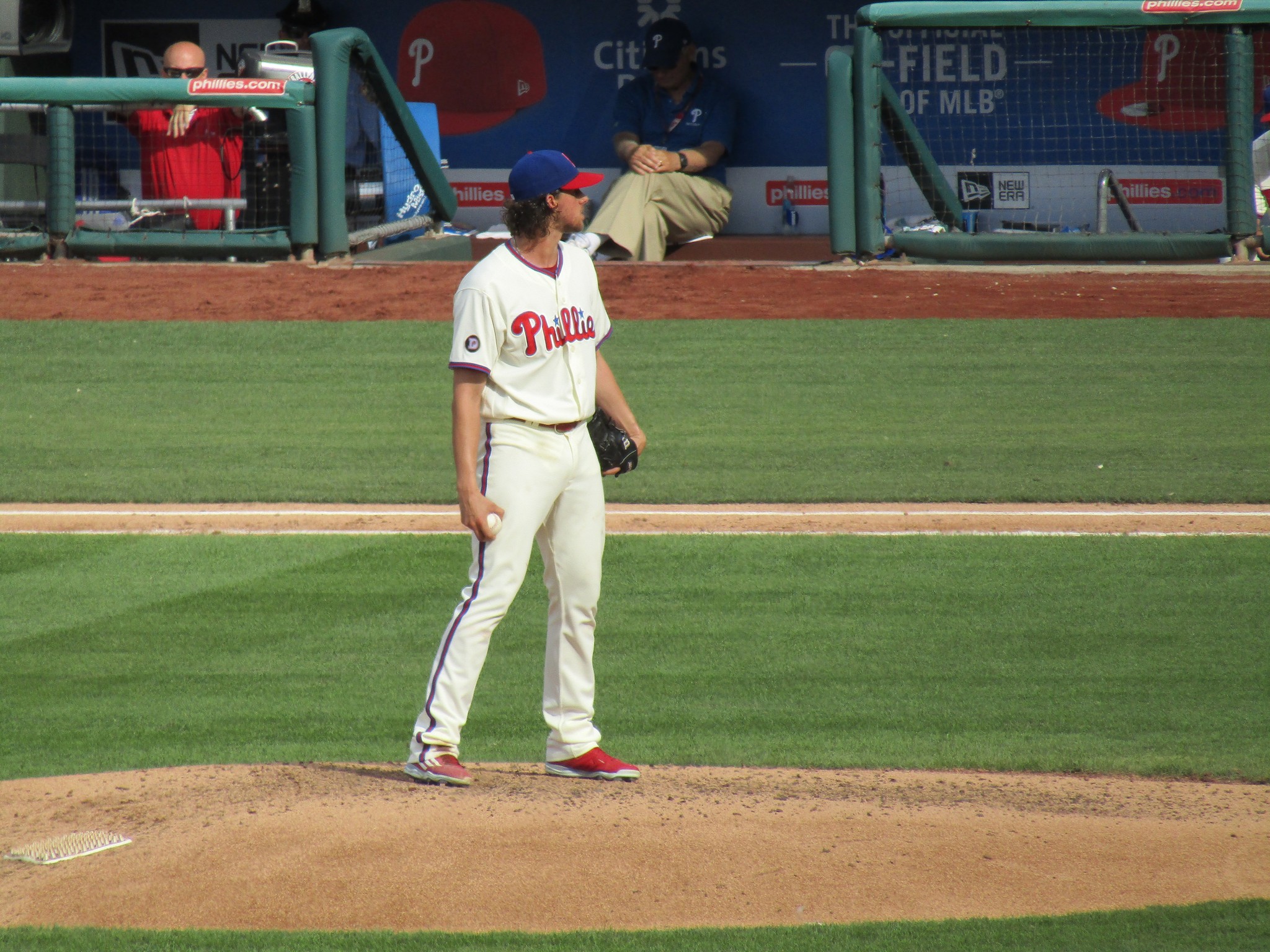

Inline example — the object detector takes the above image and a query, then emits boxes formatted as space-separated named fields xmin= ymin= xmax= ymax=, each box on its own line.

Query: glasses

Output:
xmin=163 ymin=66 xmax=205 ymax=79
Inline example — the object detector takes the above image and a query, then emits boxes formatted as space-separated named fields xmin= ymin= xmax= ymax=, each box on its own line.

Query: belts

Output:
xmin=513 ymin=416 xmax=580 ymax=433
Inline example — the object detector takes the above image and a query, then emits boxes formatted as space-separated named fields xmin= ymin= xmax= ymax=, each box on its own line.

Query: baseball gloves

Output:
xmin=587 ymin=407 xmax=639 ymax=478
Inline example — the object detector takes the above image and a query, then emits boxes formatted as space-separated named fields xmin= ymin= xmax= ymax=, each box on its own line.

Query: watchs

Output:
xmin=676 ymin=152 xmax=688 ymax=171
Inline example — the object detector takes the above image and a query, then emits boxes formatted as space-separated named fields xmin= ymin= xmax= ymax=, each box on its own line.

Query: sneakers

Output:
xmin=545 ymin=747 xmax=638 ymax=780
xmin=404 ymin=754 xmax=473 ymax=785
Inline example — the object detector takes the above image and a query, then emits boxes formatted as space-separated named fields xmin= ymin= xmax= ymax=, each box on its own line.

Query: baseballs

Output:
xmin=486 ymin=512 xmax=502 ymax=537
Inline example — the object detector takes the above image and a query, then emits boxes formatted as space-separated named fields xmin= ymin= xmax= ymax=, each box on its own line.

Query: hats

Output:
xmin=510 ymin=149 xmax=607 ymax=198
xmin=642 ymin=16 xmax=691 ymax=67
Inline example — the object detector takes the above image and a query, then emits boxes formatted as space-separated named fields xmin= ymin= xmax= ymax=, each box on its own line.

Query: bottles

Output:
xmin=782 ymin=174 xmax=799 ymax=238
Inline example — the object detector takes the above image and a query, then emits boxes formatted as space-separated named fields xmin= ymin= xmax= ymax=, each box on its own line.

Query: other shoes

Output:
xmin=563 ymin=233 xmax=596 ymax=261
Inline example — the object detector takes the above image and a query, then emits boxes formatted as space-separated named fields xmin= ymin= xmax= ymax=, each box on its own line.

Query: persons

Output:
xmin=1252 ymin=85 xmax=1270 ymax=263
xmin=401 ymin=151 xmax=646 ymax=785
xmin=114 ymin=41 xmax=267 ymax=231
xmin=561 ymin=18 xmax=733 ymax=262
